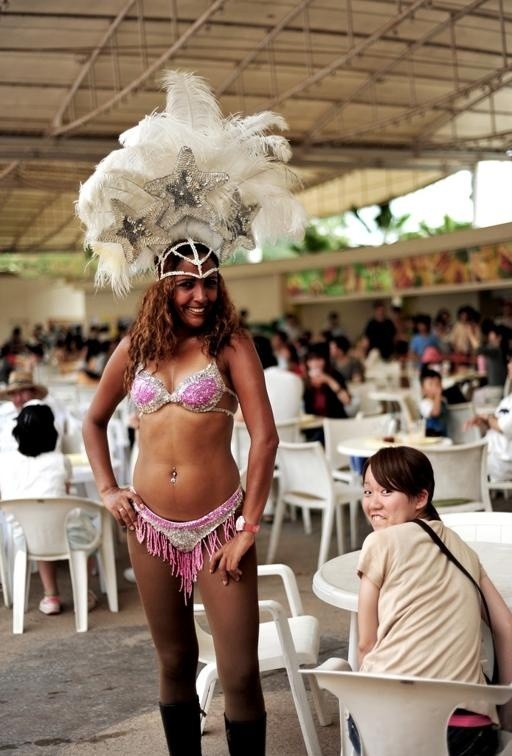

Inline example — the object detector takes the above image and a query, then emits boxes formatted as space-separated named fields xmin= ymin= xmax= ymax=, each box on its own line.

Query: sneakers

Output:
xmin=38 ymin=592 xmax=60 ymax=615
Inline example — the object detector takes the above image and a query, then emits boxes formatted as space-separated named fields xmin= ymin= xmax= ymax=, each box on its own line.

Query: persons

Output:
xmin=0 ymin=315 xmax=140 ymax=614
xmin=80 ymin=235 xmax=284 ymax=756
xmin=354 ymin=446 xmax=512 ymax=756
xmin=237 ymin=298 xmax=512 ymax=482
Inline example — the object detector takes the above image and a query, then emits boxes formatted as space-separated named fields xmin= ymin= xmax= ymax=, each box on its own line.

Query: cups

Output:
xmin=379 ymin=420 xmax=396 ymax=444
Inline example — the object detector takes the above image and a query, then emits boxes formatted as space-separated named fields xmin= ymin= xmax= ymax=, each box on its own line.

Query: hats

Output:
xmin=0 ymin=370 xmax=49 ymax=404
